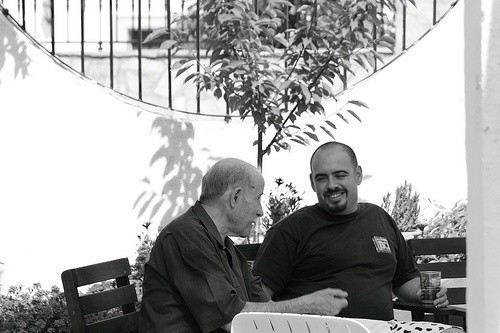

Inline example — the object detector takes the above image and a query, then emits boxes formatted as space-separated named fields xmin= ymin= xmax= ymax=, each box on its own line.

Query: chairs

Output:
xmin=405 ymin=237 xmax=466 ymax=332
xmin=231 ymin=311 xmax=369 ymax=333
xmin=61 ymin=257 xmax=140 ymax=332
xmin=233 ymin=243 xmax=452 ymax=324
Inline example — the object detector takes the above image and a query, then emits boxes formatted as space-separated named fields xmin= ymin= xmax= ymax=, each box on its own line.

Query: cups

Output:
xmin=420 ymin=271 xmax=441 ymax=305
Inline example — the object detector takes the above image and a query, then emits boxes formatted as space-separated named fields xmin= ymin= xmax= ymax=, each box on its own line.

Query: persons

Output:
xmin=137 ymin=158 xmax=348 ymax=333
xmin=252 ymin=141 xmax=466 ymax=333
